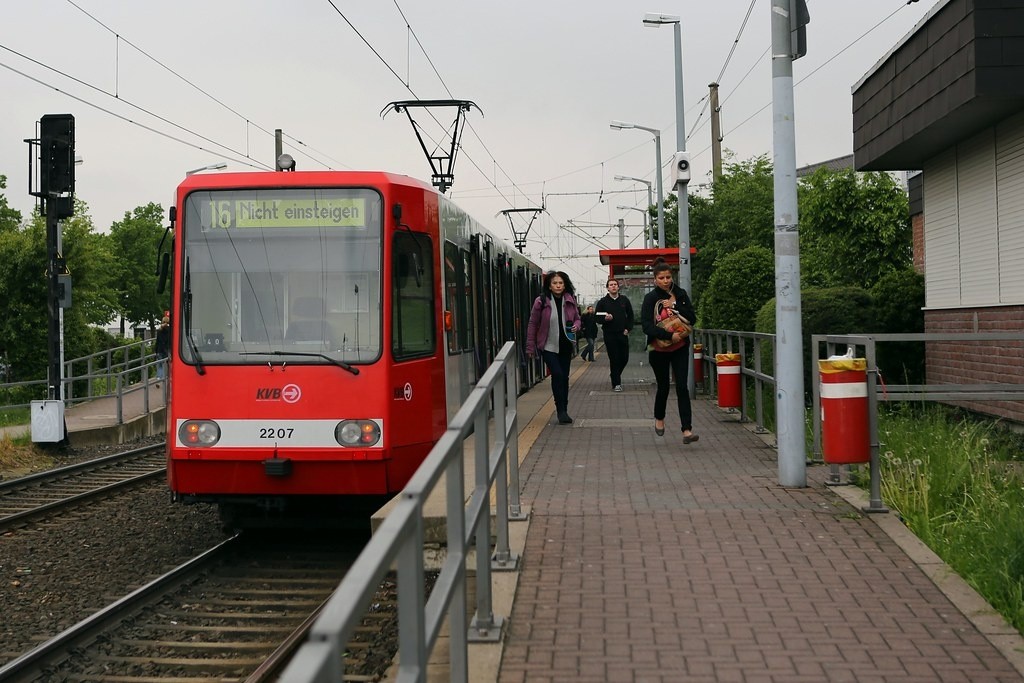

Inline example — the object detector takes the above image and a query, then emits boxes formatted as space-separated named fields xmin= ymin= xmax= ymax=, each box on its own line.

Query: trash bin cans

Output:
xmin=818 ymin=358 xmax=871 ymax=464
xmin=693 ymin=343 xmax=703 ymax=382
xmin=715 ymin=353 xmax=742 ymax=407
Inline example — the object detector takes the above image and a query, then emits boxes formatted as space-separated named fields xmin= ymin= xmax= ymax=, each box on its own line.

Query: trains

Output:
xmin=164 ymin=151 xmax=551 ymax=504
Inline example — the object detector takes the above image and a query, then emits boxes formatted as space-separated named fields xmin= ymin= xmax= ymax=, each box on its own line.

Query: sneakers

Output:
xmin=609 ymin=373 xmax=611 ymax=378
xmin=614 ymin=385 xmax=623 ymax=392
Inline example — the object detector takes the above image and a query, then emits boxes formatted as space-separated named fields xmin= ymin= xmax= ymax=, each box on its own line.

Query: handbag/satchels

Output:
xmin=653 ymin=299 xmax=693 ymax=349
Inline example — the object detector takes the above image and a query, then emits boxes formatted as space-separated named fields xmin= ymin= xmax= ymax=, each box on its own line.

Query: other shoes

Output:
xmin=581 ymin=355 xmax=587 ymax=361
xmin=588 ymin=359 xmax=597 ymax=362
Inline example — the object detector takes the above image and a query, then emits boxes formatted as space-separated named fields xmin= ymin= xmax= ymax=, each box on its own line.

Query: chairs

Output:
xmin=283 ymin=296 xmax=333 ymax=352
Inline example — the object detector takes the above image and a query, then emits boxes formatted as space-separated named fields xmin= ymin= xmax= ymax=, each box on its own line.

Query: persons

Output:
xmin=580 ymin=306 xmax=599 ymax=362
xmin=594 ymin=279 xmax=635 ymax=392
xmin=152 ymin=311 xmax=171 ymax=407
xmin=641 ymin=257 xmax=699 ymax=445
xmin=525 ymin=270 xmax=582 ymax=424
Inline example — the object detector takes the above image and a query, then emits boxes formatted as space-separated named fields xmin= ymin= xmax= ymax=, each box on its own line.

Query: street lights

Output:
xmin=643 ymin=14 xmax=695 ymax=394
xmin=610 ymin=119 xmax=665 ymax=248
xmin=614 ymin=174 xmax=654 ymax=249
xmin=185 ymin=162 xmax=227 ymax=176
xmin=617 ymin=205 xmax=647 ymax=249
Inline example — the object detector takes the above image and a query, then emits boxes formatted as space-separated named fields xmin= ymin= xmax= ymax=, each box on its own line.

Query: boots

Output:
xmin=556 ymin=403 xmax=573 ymax=424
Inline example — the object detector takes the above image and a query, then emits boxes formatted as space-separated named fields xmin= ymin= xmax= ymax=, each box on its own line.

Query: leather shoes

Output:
xmin=683 ymin=434 xmax=699 ymax=444
xmin=654 ymin=419 xmax=665 ymax=437
